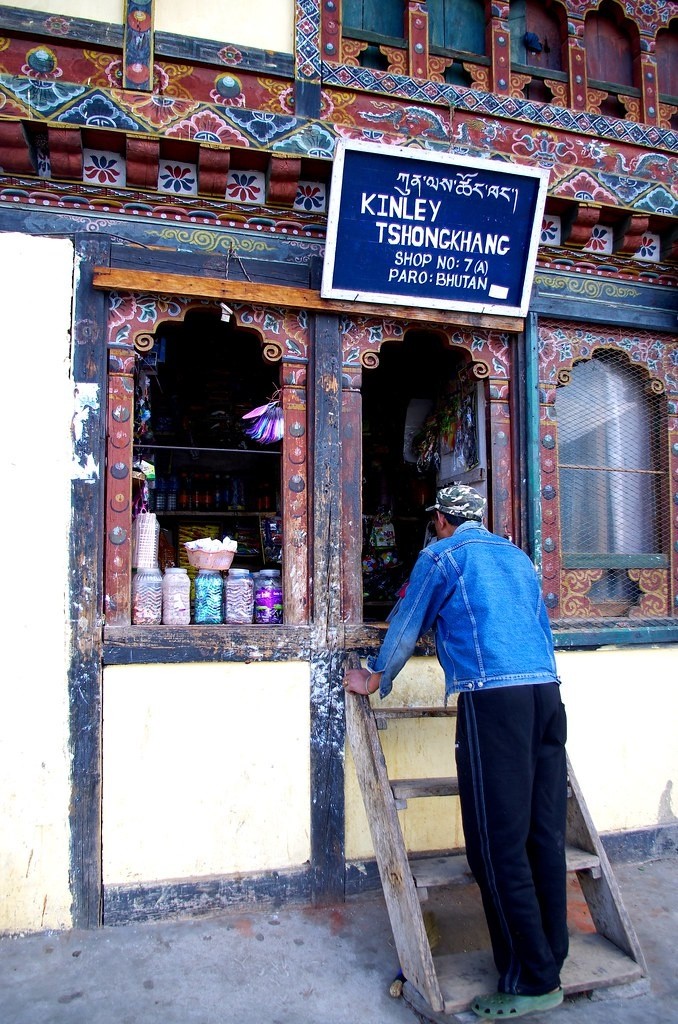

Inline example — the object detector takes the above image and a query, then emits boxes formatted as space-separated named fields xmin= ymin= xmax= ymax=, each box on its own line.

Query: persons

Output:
xmin=343 ymin=485 xmax=569 ymax=1019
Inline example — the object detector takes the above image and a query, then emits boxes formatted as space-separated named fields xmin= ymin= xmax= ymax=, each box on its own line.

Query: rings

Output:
xmin=344 ymin=680 xmax=348 ymax=687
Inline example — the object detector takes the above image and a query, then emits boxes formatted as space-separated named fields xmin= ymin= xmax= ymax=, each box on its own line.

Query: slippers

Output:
xmin=471 ymin=983 xmax=564 ymax=1019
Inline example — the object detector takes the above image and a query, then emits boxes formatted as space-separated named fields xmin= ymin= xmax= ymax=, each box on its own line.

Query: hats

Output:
xmin=425 ymin=483 xmax=484 ymax=521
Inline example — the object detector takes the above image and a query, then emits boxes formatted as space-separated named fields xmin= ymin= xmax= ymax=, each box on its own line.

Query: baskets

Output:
xmin=185 ymin=546 xmax=236 ymax=570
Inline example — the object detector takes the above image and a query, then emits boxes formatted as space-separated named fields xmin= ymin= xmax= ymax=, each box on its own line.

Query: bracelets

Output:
xmin=365 ymin=676 xmax=372 ymax=695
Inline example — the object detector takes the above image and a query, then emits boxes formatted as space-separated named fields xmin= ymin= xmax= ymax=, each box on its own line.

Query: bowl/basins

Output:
xmin=185 ymin=546 xmax=237 ymax=570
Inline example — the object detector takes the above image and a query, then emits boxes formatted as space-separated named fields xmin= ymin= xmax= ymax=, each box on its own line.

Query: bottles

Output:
xmin=194 ymin=570 xmax=223 ymax=625
xmin=163 ymin=568 xmax=191 ymax=625
xmin=224 ymin=568 xmax=254 ymax=624
xmin=254 ymin=570 xmax=283 ymax=624
xmin=131 ymin=513 xmax=160 ymax=568
xmin=132 ymin=568 xmax=163 ymax=625
xmin=153 ymin=469 xmax=282 ymax=512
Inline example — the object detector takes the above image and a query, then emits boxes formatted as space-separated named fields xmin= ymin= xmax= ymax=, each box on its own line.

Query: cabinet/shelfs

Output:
xmin=128 ymin=306 xmax=490 ymax=632
